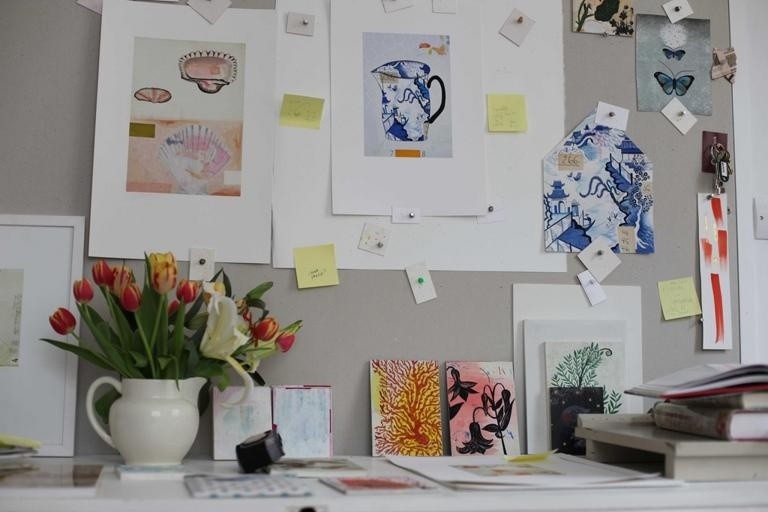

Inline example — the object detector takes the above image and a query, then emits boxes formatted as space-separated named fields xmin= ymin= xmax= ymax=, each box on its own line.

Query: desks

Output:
xmin=0 ymin=448 xmax=766 ymax=507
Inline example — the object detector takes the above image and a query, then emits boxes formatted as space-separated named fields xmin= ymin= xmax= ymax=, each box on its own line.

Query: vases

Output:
xmin=81 ymin=376 xmax=209 ymax=466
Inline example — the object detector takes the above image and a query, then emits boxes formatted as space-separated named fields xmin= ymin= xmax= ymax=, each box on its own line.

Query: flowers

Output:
xmin=34 ymin=249 xmax=306 ymax=408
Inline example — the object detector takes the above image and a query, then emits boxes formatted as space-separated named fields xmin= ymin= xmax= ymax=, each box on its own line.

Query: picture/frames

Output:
xmin=0 ymin=215 xmax=88 ymax=458
